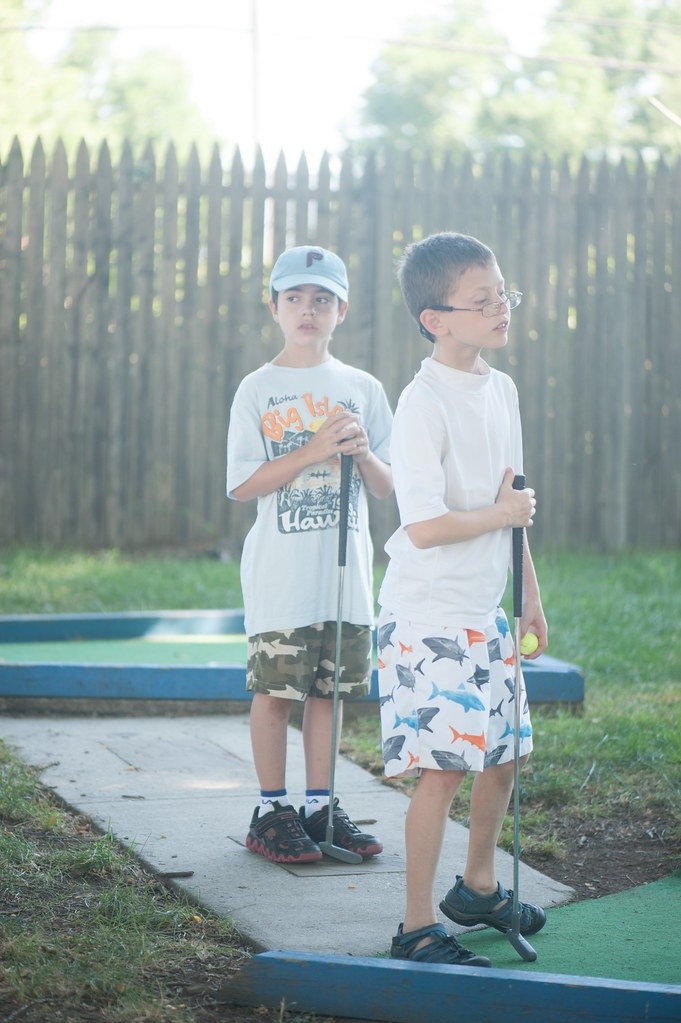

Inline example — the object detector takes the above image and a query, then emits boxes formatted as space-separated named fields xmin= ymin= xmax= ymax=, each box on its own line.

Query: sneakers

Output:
xmin=440 ymin=874 xmax=546 ymax=934
xmin=298 ymin=797 xmax=384 ymax=857
xmin=246 ymin=801 xmax=324 ymax=863
xmin=391 ymin=920 xmax=491 ymax=968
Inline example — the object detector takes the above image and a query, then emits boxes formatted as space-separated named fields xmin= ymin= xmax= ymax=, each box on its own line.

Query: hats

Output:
xmin=270 ymin=245 xmax=349 ymax=304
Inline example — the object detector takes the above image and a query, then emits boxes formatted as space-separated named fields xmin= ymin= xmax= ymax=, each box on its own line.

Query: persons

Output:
xmin=227 ymin=245 xmax=395 ymax=866
xmin=375 ymin=229 xmax=549 ymax=970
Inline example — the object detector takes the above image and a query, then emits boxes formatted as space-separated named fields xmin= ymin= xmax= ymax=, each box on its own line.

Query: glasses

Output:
xmin=426 ymin=291 xmax=523 ymax=319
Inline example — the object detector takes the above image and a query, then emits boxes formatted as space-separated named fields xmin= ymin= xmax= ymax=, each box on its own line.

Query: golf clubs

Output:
xmin=501 ymin=474 xmax=540 ymax=963
xmin=312 ymin=435 xmax=367 ymax=867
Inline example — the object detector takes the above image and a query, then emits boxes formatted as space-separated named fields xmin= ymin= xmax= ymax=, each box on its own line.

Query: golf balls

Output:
xmin=516 ymin=630 xmax=540 ymax=658
